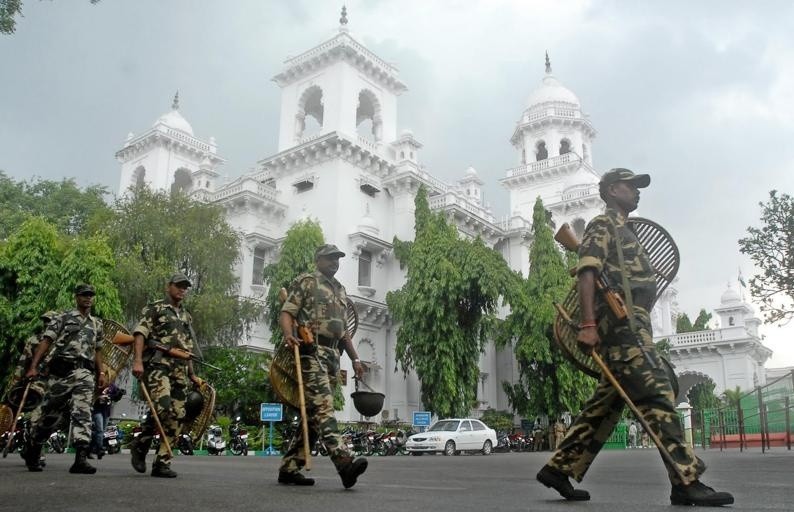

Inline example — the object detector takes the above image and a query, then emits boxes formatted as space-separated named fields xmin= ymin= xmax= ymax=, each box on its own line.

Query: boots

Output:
xmin=19 ymin=440 xmax=104 ymax=474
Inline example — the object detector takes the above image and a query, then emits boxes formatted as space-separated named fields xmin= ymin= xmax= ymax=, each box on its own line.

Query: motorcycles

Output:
xmin=493 ymin=428 xmax=534 ymax=452
xmin=0 ymin=412 xmax=250 ymax=457
xmin=278 ymin=414 xmax=425 ymax=459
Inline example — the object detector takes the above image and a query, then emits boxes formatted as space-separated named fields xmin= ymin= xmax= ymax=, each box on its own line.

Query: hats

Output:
xmin=167 ymin=273 xmax=191 ymax=287
xmin=37 ymin=311 xmax=60 ymax=320
xmin=313 ymin=245 xmax=344 ymax=263
xmin=76 ymin=285 xmax=95 ymax=295
xmin=599 ymin=168 xmax=650 ymax=188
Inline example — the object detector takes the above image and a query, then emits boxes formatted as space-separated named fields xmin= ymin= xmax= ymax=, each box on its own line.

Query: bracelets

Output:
xmin=352 ymin=359 xmax=360 ymax=363
xmin=187 ymin=372 xmax=194 ymax=377
xmin=579 ymin=320 xmax=597 ymax=329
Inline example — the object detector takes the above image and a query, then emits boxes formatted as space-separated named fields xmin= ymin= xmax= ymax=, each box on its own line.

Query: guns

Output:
xmin=278 ymin=288 xmax=325 ymax=374
xmin=552 ymin=222 xmax=657 ymax=369
xmin=113 ymin=330 xmax=222 ymax=372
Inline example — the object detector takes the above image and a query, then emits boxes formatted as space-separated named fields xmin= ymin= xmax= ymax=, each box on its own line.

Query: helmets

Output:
xmin=183 ymin=388 xmax=204 ymax=417
xmin=661 ymin=356 xmax=679 ymax=396
xmin=350 ymin=391 xmax=384 ymax=417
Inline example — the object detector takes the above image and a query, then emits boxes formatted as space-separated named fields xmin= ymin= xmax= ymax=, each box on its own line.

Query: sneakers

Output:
xmin=130 ymin=446 xmax=147 ymax=472
xmin=537 ymin=463 xmax=590 ymax=501
xmin=277 ymin=470 xmax=314 ymax=486
xmin=337 ymin=458 xmax=367 ymax=488
xmin=150 ymin=463 xmax=177 ymax=477
xmin=668 ymin=478 xmax=734 ymax=506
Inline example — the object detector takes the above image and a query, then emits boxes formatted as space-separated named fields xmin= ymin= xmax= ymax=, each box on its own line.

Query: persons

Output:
xmin=641 ymin=425 xmax=649 ymax=448
xmin=537 ymin=169 xmax=734 ymax=506
xmin=278 ymin=245 xmax=368 ymax=489
xmin=130 ymin=273 xmax=207 ymax=478
xmin=629 ymin=422 xmax=637 ymax=448
xmin=533 ymin=419 xmax=566 ymax=451
xmin=20 ymin=284 xmax=118 ymax=474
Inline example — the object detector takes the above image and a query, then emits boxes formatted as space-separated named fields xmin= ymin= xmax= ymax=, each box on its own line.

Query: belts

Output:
xmin=318 ymin=335 xmax=340 ymax=349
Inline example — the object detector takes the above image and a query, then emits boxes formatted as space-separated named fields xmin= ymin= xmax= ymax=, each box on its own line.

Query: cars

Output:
xmin=404 ymin=417 xmax=498 ymax=456
